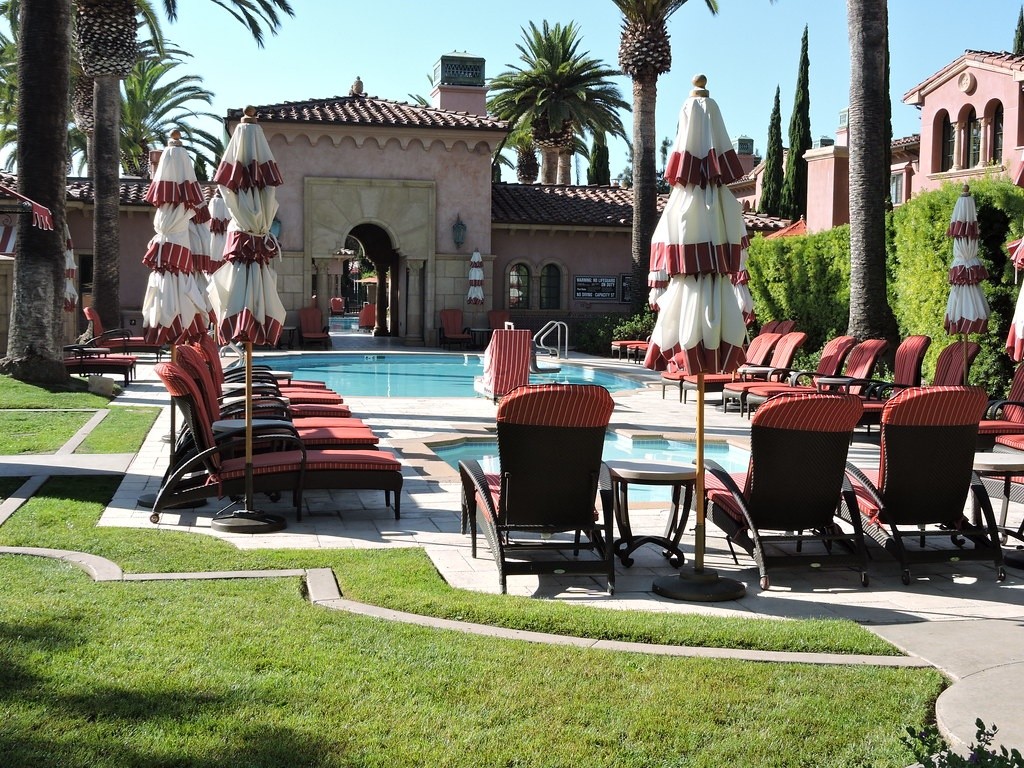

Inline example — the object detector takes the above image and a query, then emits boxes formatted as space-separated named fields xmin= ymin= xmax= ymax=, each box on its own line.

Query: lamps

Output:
xmin=269 ymin=214 xmax=281 ymax=240
xmin=453 ymin=212 xmax=466 ymax=249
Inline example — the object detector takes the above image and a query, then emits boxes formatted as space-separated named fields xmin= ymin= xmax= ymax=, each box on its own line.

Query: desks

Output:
xmin=210 ymin=418 xmax=296 ymax=502
xmin=72 ymin=347 xmax=110 ymax=378
xmin=470 ymin=328 xmax=493 ymax=351
xmin=269 ymin=327 xmax=297 ymax=350
xmin=605 ymin=459 xmax=697 ymax=569
xmin=221 ymin=382 xmax=276 ymax=392
xmin=250 ymin=370 xmax=292 ymax=387
xmin=222 ymin=396 xmax=291 ymax=407
xmin=951 ymin=453 xmax=1024 ymax=547
xmin=736 ymin=367 xmax=779 ymax=382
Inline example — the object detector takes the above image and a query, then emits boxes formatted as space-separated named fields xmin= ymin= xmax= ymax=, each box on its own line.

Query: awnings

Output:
xmin=0 ymin=185 xmax=54 ymax=230
xmin=0 ymin=226 xmax=18 ymax=253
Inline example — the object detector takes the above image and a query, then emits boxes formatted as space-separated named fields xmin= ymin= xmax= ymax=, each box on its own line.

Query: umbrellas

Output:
xmin=648 ymin=271 xmax=670 ymax=312
xmin=205 ymin=188 xmax=232 ymax=278
xmin=206 ymin=107 xmax=287 ymax=510
xmin=62 ymin=226 xmax=78 ymax=312
xmin=467 ymin=249 xmax=484 ymax=329
xmin=729 ymin=252 xmax=755 ymax=325
xmin=141 ymin=128 xmax=218 ymax=470
xmin=644 ymin=73 xmax=750 ymax=570
xmin=944 ymin=184 xmax=991 ymax=385
xmin=1004 ymin=236 xmax=1024 ymax=364
xmin=509 ymin=266 xmax=523 ymax=307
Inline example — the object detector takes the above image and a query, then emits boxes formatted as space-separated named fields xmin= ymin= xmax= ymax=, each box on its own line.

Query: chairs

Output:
xmin=661 ymin=319 xmax=1024 ymax=591
xmin=817 ymin=378 xmax=855 ymax=394
xmin=612 ymin=333 xmax=651 ymax=364
xmin=457 ymin=382 xmax=616 ymax=596
xmin=474 ymin=329 xmax=532 ymax=405
xmin=149 ymin=331 xmax=404 ymax=524
xmin=329 ymin=298 xmax=346 ymax=318
xmin=297 ymin=308 xmax=330 ymax=351
xmin=60 ymin=305 xmax=162 ymax=387
xmin=358 ymin=303 xmax=375 ymax=333
xmin=439 ymin=309 xmax=473 ymax=352
xmin=487 ymin=310 xmax=510 ymax=345
